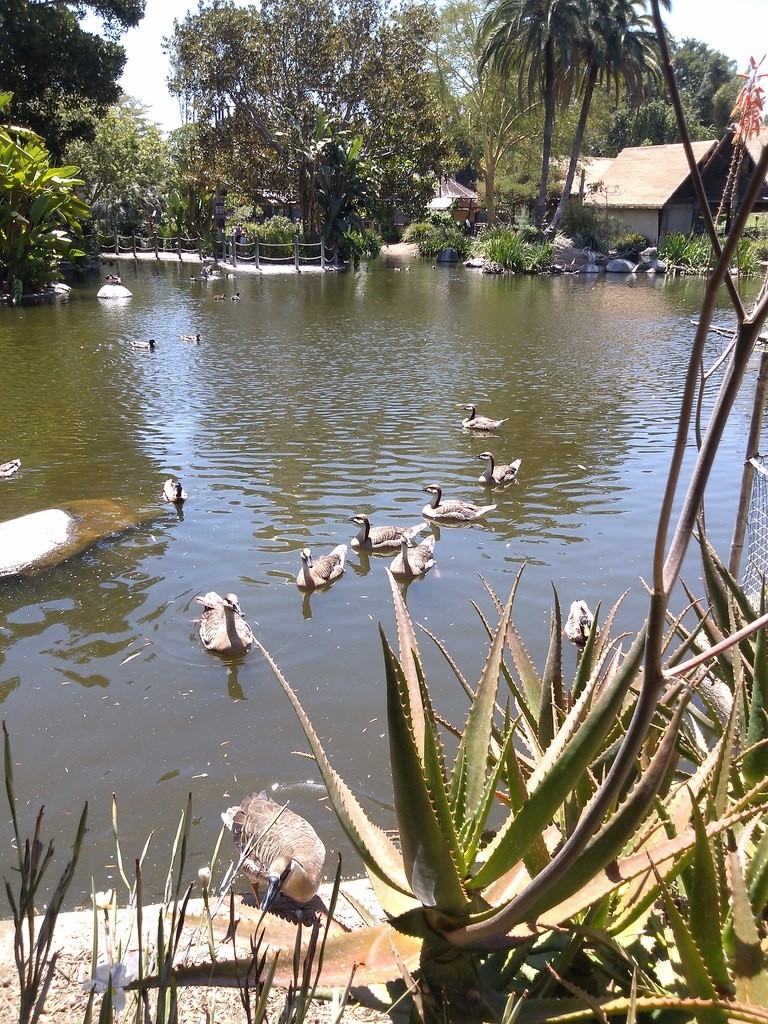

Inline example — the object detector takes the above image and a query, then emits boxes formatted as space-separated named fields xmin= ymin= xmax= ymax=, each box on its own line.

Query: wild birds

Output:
xmin=219 ymin=787 xmax=328 ymax=913
xmin=563 ymin=599 xmax=601 ymax=646
xmin=188 ymin=590 xmax=255 ymax=652
xmin=104 ymin=264 xmax=241 ymax=350
xmin=162 ymin=477 xmax=189 ymax=503
xmin=295 ymin=402 xmax=523 ymax=588
xmin=383 ymin=243 xmax=437 ymax=273
xmin=0 ymin=458 xmax=22 ymax=478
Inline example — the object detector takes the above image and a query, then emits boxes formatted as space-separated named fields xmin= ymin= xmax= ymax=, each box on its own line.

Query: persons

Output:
xmin=235 ymin=223 xmax=247 ymax=261
